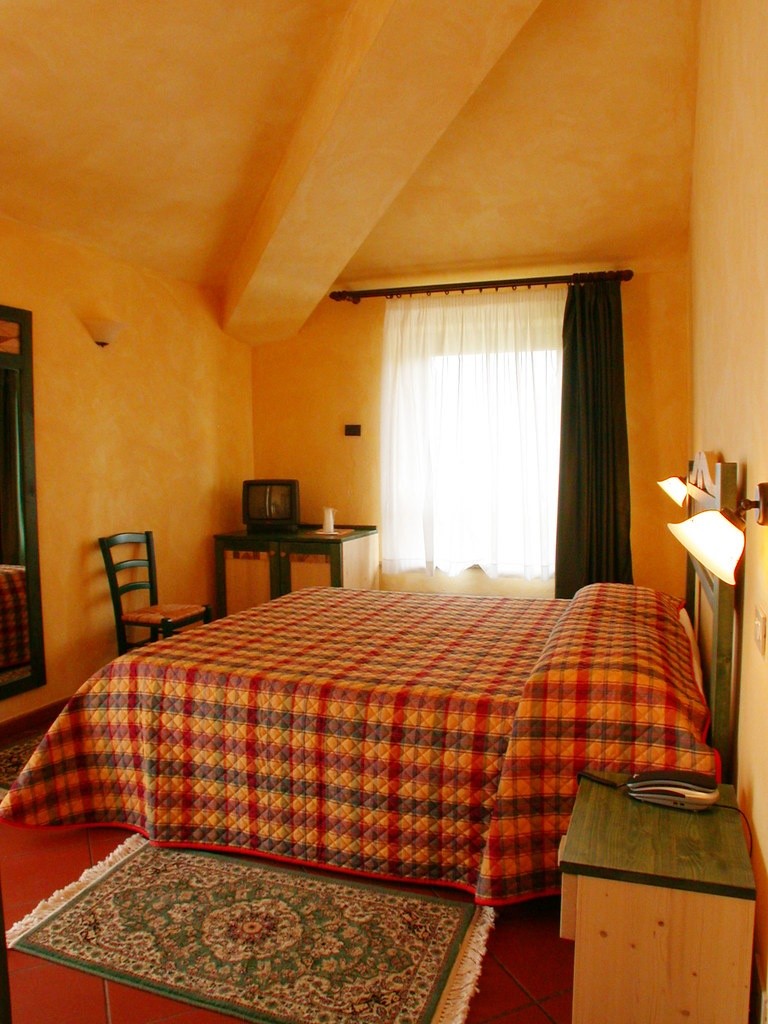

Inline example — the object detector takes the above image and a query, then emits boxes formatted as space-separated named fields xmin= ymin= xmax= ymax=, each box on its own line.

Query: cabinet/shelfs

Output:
xmin=214 ymin=522 xmax=380 ymax=619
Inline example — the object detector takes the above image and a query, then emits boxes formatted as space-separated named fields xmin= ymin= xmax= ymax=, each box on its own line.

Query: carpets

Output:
xmin=5 ymin=833 xmax=496 ymax=1024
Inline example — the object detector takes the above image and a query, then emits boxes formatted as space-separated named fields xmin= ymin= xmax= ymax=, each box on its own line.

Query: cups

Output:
xmin=323 ymin=509 xmax=334 ymax=533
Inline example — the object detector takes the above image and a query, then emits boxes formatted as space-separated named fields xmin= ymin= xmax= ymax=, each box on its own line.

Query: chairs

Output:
xmin=97 ymin=531 xmax=212 ymax=657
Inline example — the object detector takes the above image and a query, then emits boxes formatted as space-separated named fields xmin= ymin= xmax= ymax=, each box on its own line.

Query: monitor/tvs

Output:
xmin=243 ymin=480 xmax=300 ymax=534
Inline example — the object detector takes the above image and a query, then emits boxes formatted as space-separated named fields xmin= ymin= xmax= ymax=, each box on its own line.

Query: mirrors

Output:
xmin=0 ymin=305 xmax=47 ymax=699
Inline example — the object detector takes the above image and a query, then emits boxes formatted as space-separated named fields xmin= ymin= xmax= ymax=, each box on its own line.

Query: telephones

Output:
xmin=626 ymin=770 xmax=720 ymax=811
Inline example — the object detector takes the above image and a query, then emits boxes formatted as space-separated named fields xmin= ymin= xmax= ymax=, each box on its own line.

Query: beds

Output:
xmin=0 ymin=449 xmax=738 ymax=905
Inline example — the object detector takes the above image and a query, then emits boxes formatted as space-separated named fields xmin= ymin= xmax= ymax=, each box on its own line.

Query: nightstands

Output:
xmin=559 ymin=769 xmax=756 ymax=1024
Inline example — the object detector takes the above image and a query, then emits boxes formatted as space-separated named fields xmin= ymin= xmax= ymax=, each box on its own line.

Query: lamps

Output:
xmin=657 ymin=476 xmax=688 ymax=508
xmin=668 ymin=482 xmax=768 ymax=586
xmin=82 ymin=318 xmax=128 ymax=348
